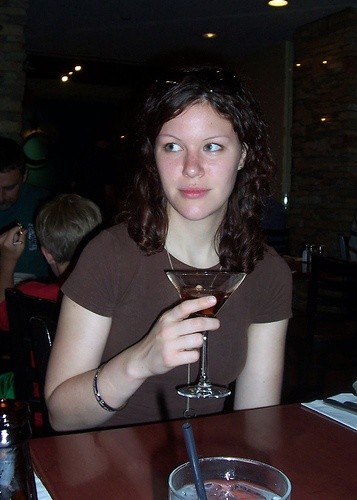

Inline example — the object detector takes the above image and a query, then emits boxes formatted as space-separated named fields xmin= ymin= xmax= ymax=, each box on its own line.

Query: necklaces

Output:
xmin=165 ymin=245 xmax=221 ymax=417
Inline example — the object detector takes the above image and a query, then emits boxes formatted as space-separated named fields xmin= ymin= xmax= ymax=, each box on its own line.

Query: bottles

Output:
xmin=302 ymin=242 xmax=327 ymax=263
xmin=0 ymin=397 xmax=39 ymax=500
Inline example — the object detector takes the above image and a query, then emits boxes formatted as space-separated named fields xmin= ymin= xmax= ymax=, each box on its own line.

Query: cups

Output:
xmin=167 ymin=456 xmax=292 ymax=500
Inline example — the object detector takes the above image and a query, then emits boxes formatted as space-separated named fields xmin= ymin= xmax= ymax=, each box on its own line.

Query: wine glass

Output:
xmin=166 ymin=270 xmax=247 ymax=400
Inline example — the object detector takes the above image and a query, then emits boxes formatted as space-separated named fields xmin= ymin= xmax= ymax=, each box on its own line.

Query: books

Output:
xmin=301 ymin=393 xmax=357 ymax=431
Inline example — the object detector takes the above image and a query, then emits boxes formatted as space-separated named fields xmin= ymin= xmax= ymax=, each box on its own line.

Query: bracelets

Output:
xmin=93 ymin=364 xmax=128 ymax=412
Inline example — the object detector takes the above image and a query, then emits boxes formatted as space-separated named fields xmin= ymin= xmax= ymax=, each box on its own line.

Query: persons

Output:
xmin=0 ymin=136 xmax=47 ymax=233
xmin=0 ymin=195 xmax=103 ymax=426
xmin=44 ymin=68 xmax=293 ymax=430
xmin=348 ymin=213 xmax=357 ymax=262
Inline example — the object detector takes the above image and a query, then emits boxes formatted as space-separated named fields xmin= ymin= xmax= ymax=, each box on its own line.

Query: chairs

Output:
xmin=282 ymin=252 xmax=357 ymax=404
xmin=5 ymin=287 xmax=63 ymax=439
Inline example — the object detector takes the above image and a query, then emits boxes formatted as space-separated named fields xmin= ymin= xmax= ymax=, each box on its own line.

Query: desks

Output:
xmin=278 ymin=255 xmax=347 ymax=316
xmin=28 ymin=402 xmax=357 ymax=500
